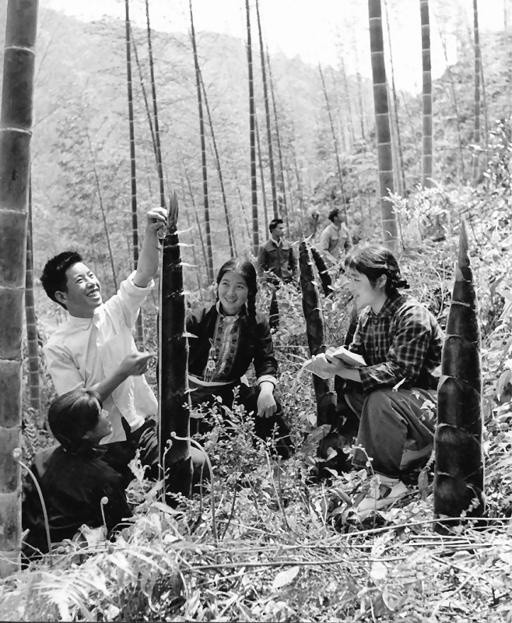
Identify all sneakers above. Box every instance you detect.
[341,477,408,520]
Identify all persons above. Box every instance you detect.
[312,245,446,512]
[41,207,211,500]
[320,207,353,265]
[257,219,298,283]
[185,257,296,460]
[21,389,133,569]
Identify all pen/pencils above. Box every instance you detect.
[337,345,348,349]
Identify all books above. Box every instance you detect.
[302,348,367,380]
